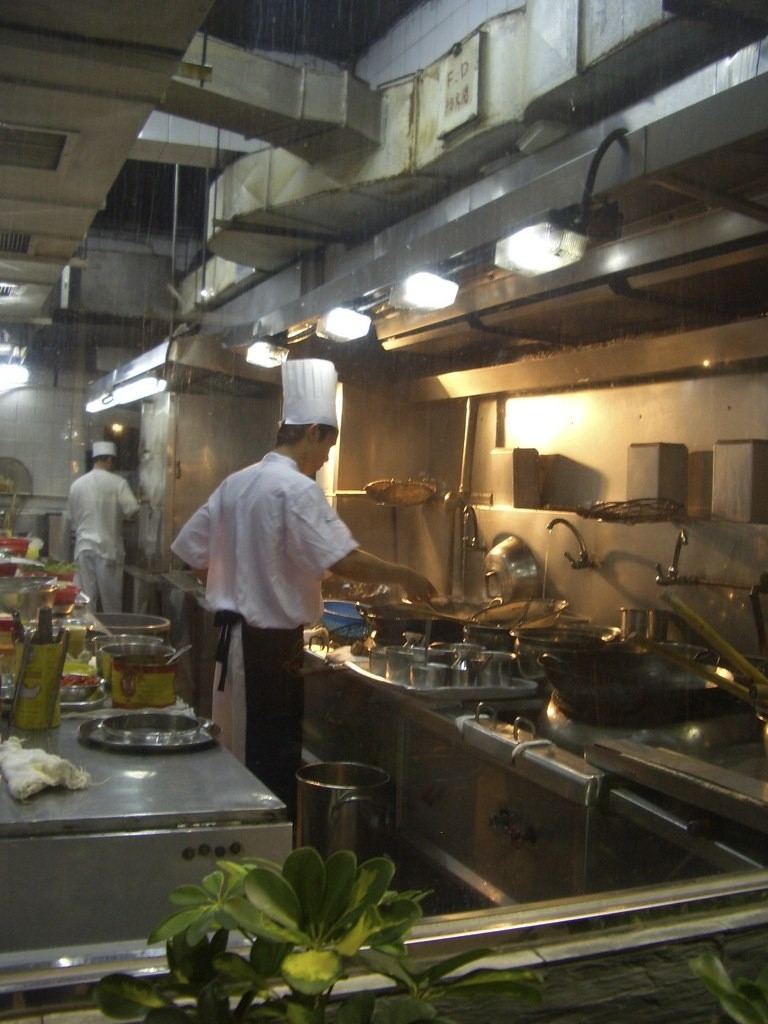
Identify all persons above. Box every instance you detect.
[169,358,437,852]
[65,442,140,613]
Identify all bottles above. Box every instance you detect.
[14,635,63,732]
[93,635,179,707]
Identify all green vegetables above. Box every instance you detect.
[0,550,80,574]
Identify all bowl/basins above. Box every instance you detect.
[368,643,518,689]
[0,536,75,582]
[481,531,538,605]
[58,675,107,700]
[0,575,79,618]
[319,597,365,635]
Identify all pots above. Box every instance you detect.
[461,596,621,679]
[346,595,502,640]
[535,641,719,721]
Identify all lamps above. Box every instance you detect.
[494,203,591,277]
[245,330,290,369]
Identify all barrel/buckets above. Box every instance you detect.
[295,761,391,866]
[91,610,171,646]
[619,607,669,643]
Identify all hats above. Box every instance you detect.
[279,359,339,428]
[92,442,116,458]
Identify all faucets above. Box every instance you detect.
[546,518,594,570]
[138,484,151,505]
[654,528,688,586]
[462,505,487,552]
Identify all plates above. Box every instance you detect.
[56,693,110,709]
[75,712,219,750]
